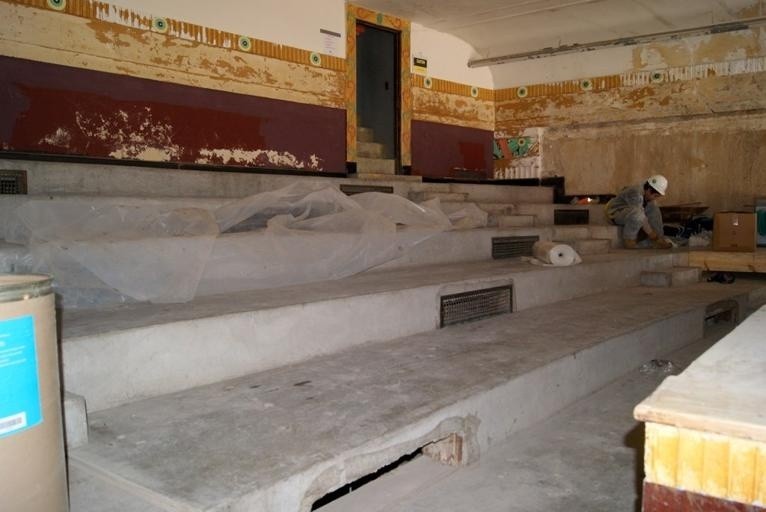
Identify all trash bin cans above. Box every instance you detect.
[756,197,766,248]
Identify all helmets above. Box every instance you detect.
[646,173,668,197]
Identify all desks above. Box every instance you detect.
[629,303,766,511]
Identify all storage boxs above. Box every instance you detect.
[712,210,758,253]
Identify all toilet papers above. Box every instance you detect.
[533,240,581,266]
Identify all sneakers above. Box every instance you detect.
[622,239,640,250]
[651,239,672,249]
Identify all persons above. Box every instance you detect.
[603,174,677,249]
